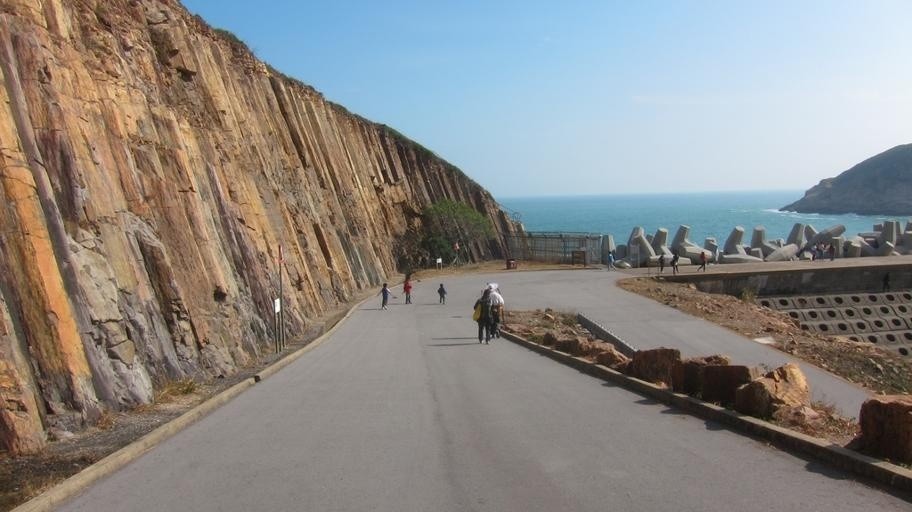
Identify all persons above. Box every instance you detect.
[437,283,447,304]
[671,256,679,275]
[473,281,506,344]
[659,251,666,273]
[402,279,413,304]
[607,251,616,272]
[810,241,835,262]
[375,282,393,310]
[696,251,707,272]
[882,271,890,292]
[673,253,681,273]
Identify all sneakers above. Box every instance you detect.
[479,330,500,345]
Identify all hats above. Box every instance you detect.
[486,283,498,291]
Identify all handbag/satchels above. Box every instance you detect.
[472,304,482,321]
